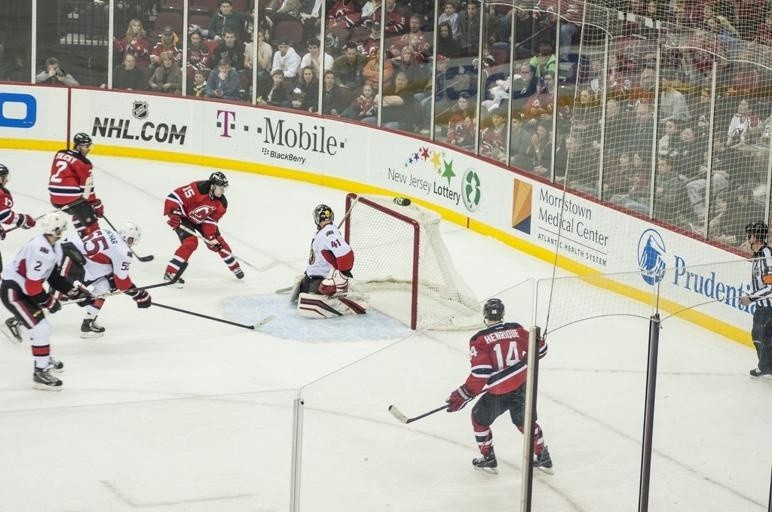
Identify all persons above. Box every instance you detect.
[294,202,370,318]
[1,130,155,391]
[445,298,554,468]
[162,171,245,283]
[738,220,771,378]
[0,0,771,254]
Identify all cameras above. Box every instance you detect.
[56,67,64,77]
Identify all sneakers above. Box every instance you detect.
[750,368,772,377]
[49,356,63,369]
[164,273,185,283]
[533,448,552,468]
[6,318,23,342]
[33,371,62,386]
[81,320,105,333]
[234,267,244,279]
[472,454,498,467]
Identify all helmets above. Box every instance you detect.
[314,205,334,227]
[118,221,141,248]
[0,164,9,176]
[209,172,228,186]
[74,132,92,146]
[40,213,69,239]
[746,221,768,242]
[483,299,504,321]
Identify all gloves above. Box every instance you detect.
[93,204,104,218]
[446,384,474,412]
[132,290,151,308]
[167,212,181,228]
[71,287,95,307]
[206,236,223,252]
[45,296,61,313]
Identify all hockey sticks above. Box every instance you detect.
[150,301,276,330]
[59,263,188,306]
[276,194,361,292]
[179,224,285,271]
[388,390,487,423]
[5,176,92,234]
[102,215,154,262]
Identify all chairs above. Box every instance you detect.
[152,0,380,91]
